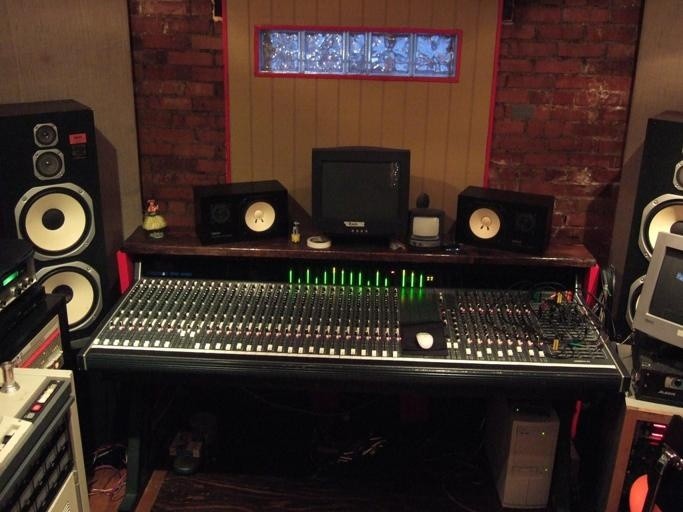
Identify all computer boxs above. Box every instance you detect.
[486,400,561,509]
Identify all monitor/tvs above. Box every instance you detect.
[633,233,683,348]
[312,147,410,235]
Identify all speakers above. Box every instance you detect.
[0,99,119,351]
[194,180,288,245]
[456,186,555,256]
[618,110,683,340]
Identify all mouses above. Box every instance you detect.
[415,332,434,349]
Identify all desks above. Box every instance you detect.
[600,380,683,512]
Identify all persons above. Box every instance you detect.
[142,199,169,238]
